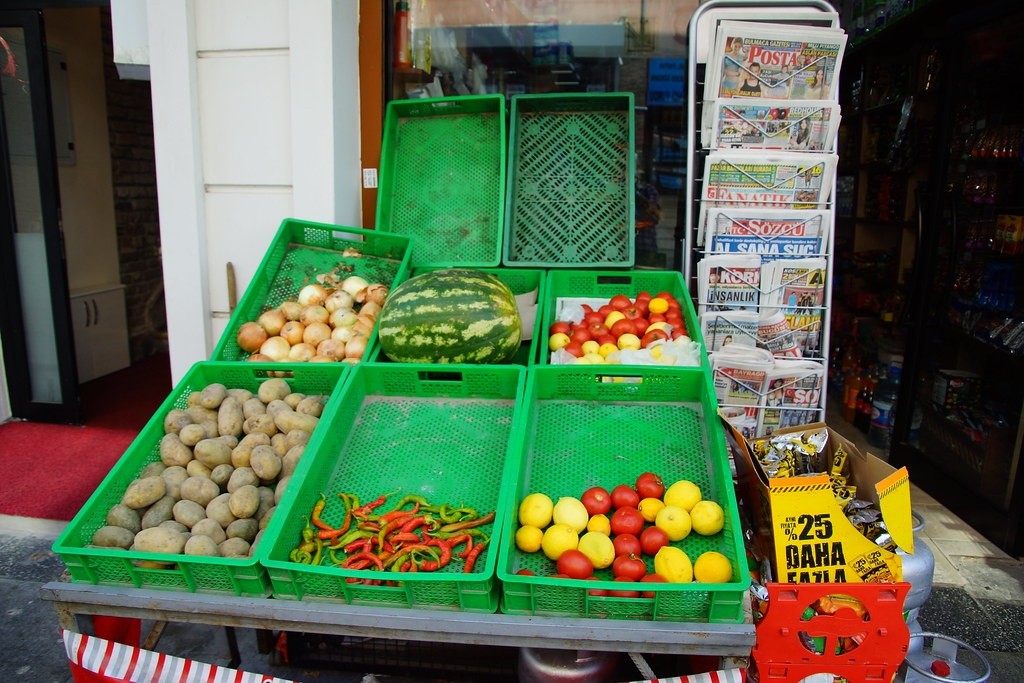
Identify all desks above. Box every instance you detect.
[39,568,756,683]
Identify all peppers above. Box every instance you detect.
[289,494,496,588]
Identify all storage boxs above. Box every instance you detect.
[718,412,914,614]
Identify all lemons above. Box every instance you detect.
[551,297,691,383]
[516,480,733,584]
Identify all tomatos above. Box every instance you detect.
[548,292,690,348]
[516,473,670,598]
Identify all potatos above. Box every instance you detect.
[92,378,323,569]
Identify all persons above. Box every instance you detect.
[710,37,829,100]
[767,378,784,406]
[710,269,720,283]
[787,292,816,315]
[794,118,810,149]
[735,426,755,439]
[723,335,732,346]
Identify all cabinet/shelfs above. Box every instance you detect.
[0,8,130,386]
[828,0,1024,563]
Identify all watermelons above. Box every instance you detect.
[378,268,522,366]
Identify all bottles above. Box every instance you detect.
[830,341,902,450]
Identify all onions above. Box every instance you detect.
[236,274,388,377]
